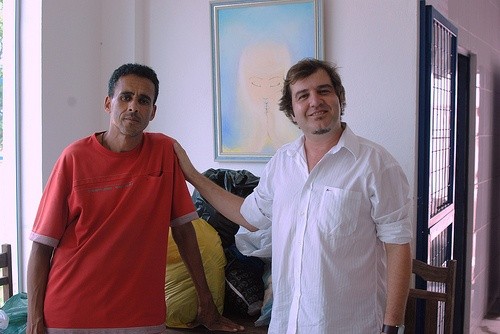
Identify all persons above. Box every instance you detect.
[173,56,414,334]
[26,64,245,334]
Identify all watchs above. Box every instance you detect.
[382,324,405,334]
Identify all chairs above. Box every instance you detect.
[405,259,457,334]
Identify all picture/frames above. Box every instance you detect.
[209,0,326,162]
